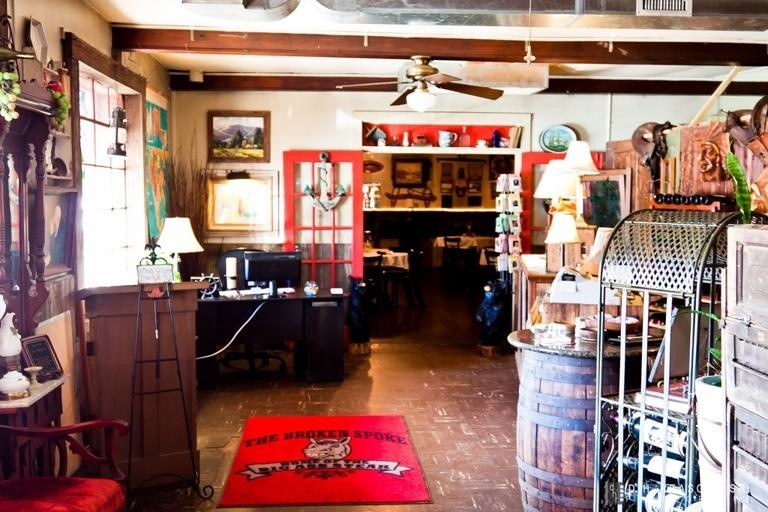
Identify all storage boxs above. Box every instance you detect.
[724,224,767,511]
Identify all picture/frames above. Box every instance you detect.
[20,332,64,383]
[391,158,427,189]
[205,107,272,164]
[199,169,282,237]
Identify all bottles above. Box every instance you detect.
[601,410,700,512]
[403,131,410,147]
[458,126,471,146]
[304,281,319,296]
[362,231,374,253]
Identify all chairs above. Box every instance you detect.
[0,414,133,512]
[363,230,501,316]
[212,248,292,373]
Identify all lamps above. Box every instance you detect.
[530,159,584,214]
[154,216,206,275]
[404,84,438,114]
[544,208,582,273]
[561,139,604,177]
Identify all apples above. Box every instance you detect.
[49,80,63,92]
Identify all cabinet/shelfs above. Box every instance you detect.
[69,281,215,491]
[0,44,71,480]
[593,210,765,512]
[683,115,767,215]
[605,123,683,258]
[517,254,619,337]
[37,124,75,284]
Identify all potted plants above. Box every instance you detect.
[689,144,756,510]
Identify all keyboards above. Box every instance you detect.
[239,286,297,297]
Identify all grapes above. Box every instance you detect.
[48,89,71,133]
[0,70,21,123]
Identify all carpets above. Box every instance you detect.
[216,412,437,508]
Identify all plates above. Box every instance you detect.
[476,145,489,148]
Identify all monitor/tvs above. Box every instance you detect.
[243,250,303,298]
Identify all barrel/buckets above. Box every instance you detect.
[694,375,725,512]
[514,349,627,512]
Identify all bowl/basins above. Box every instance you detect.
[580,327,608,341]
[604,315,640,332]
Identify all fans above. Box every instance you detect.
[335,55,503,108]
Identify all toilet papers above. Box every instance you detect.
[225,256,239,289]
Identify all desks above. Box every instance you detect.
[190,288,353,387]
[505,320,661,511]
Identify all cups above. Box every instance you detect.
[477,139,489,145]
[439,130,458,147]
